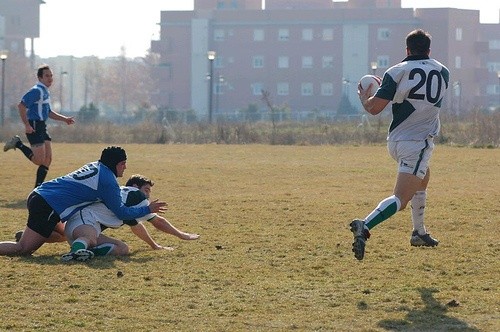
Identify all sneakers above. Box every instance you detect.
[61,249,95,261]
[15,230,23,241]
[350,219,371,260]
[4,135,22,152]
[411,230,438,247]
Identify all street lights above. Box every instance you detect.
[0,50,9,142]
[207,50,215,121]
[343,78,351,99]
[371,62,378,75]
[60,72,67,115]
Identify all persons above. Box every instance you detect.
[348,28,449,260]
[3,66,75,188]
[0,146,167,256]
[62,174,199,262]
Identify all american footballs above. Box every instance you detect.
[359,74,380,98]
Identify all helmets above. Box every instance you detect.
[98,146,127,177]
